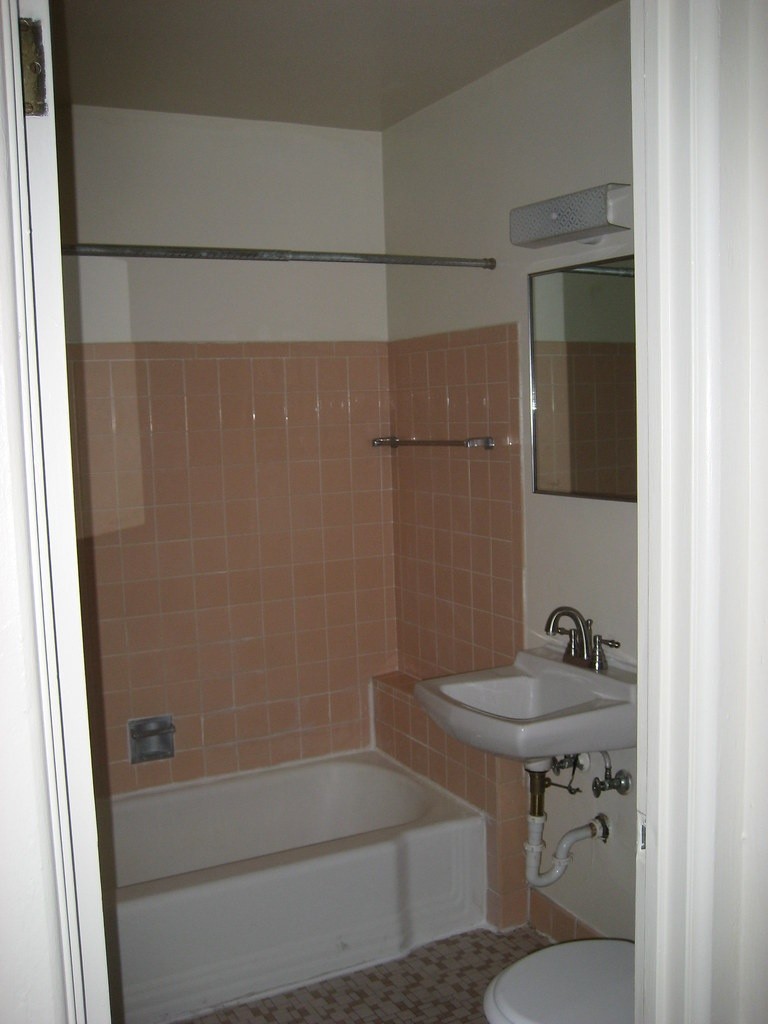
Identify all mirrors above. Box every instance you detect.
[528,254,638,505]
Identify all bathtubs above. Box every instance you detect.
[109,746,486,1024]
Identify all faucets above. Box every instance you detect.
[542,606,621,674]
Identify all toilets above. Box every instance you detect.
[487,939,634,1024]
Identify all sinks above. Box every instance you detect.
[414,645,637,762]
